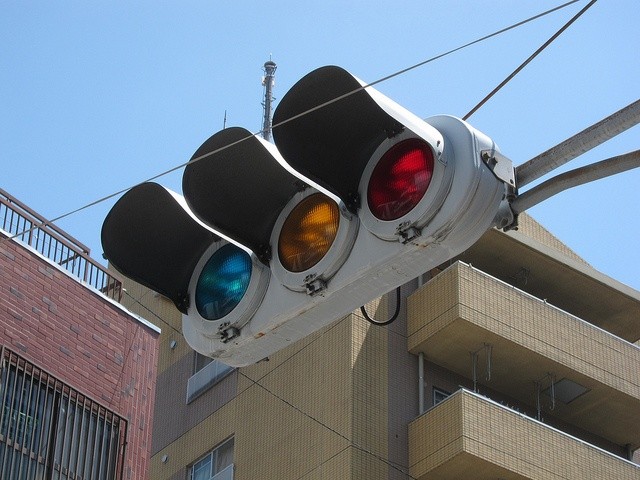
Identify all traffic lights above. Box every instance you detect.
[100,64,515,370]
